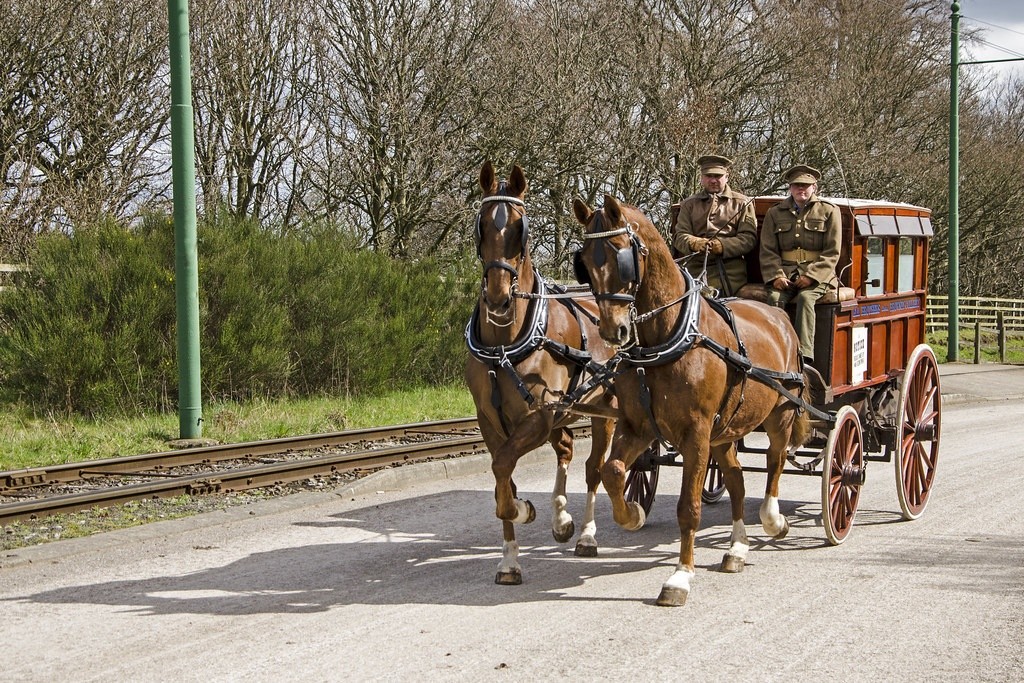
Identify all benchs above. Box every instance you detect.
[733,283,855,387]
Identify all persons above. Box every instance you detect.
[759,166,842,365]
[674,155,758,298]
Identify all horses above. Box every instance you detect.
[462,159,812,608]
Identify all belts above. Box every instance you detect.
[781,248,821,264]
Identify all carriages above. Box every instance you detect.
[461,159,944,608]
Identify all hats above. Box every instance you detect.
[785,164,821,184]
[697,156,732,175]
